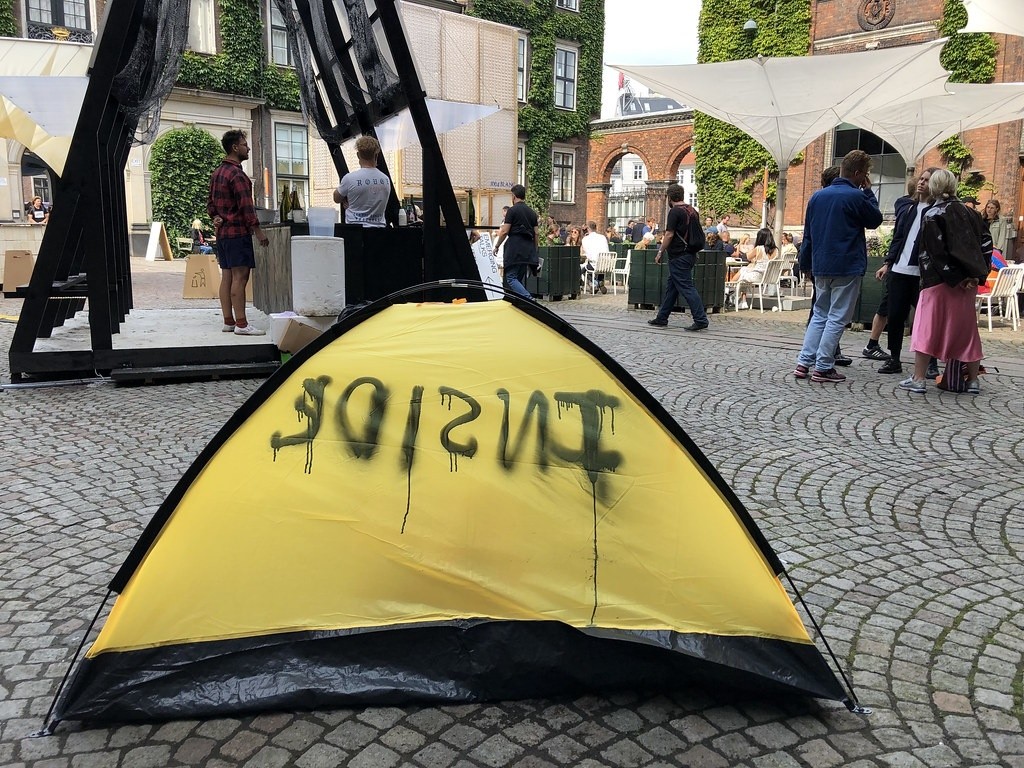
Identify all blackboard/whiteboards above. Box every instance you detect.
[146,222,173,262]
[467,232,505,300]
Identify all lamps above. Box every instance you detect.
[970,170,987,176]
[743,19,758,30]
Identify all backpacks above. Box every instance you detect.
[675,206,705,255]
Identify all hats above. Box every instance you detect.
[962,197,981,205]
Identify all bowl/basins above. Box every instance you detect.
[255,210,276,222]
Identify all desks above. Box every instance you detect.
[726,261,749,281]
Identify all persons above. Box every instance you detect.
[794,150,1007,394]
[493,184,540,303]
[333,136,391,229]
[192,218,213,254]
[206,130,269,336]
[409,205,423,222]
[538,215,667,295]
[28,195,49,225]
[500,206,510,225]
[648,184,709,331]
[702,215,797,310]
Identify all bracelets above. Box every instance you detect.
[495,246,499,248]
[882,261,890,266]
[658,250,663,253]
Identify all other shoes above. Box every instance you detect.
[591,289,599,294]
[599,281,607,294]
[738,301,748,310]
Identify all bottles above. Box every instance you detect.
[280,183,305,222]
[399,194,417,227]
[468,190,475,226]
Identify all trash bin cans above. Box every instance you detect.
[522,246,580,301]
[628,248,726,312]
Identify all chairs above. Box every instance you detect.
[976,259,1024,332]
[735,253,805,313]
[584,249,617,295]
[610,249,631,295]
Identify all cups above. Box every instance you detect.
[293,210,306,223]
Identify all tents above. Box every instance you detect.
[30,279,872,738]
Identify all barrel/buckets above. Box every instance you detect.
[307,206,335,236]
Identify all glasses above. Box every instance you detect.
[861,172,869,176]
[571,231,576,234]
[240,142,248,148]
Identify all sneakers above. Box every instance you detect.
[234,324,266,336]
[222,324,235,332]
[965,380,980,393]
[834,354,852,366]
[684,323,708,331]
[924,358,939,379]
[648,318,668,326]
[878,359,903,374]
[793,365,809,378]
[862,345,889,360]
[811,367,846,383]
[899,374,926,392]
